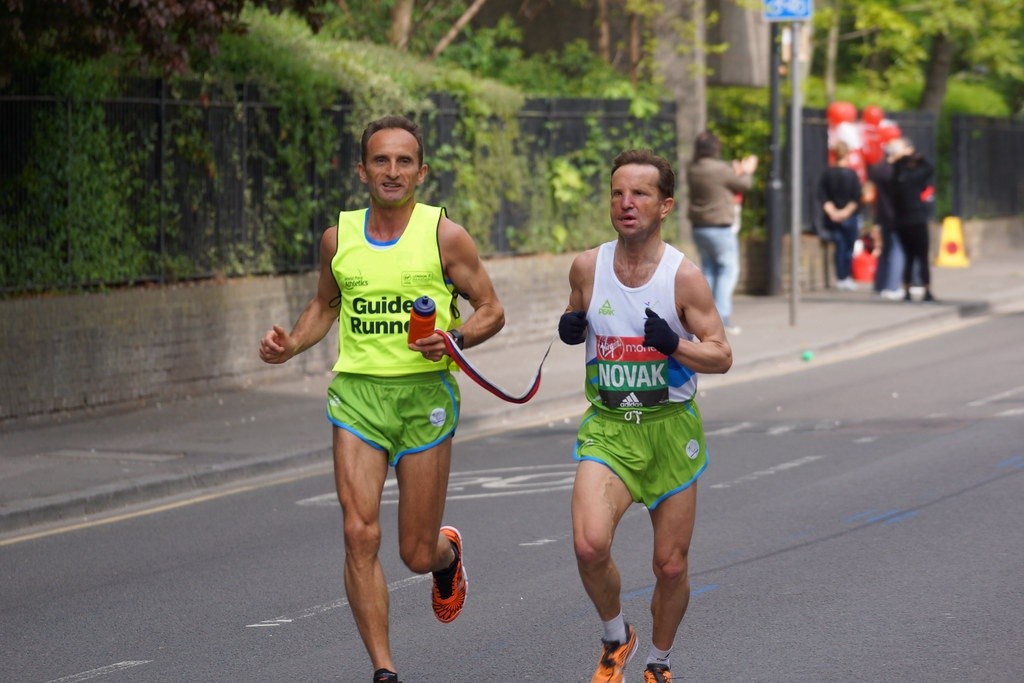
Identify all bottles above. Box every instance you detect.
[408,296,436,349]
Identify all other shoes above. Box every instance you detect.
[837,277,857,289]
[908,285,926,302]
[881,288,904,299]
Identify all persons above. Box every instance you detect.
[258,114,505,683]
[686,129,759,336]
[818,105,936,302]
[558,148,734,683]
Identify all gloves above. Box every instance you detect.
[558,311,589,345]
[641,307,679,356]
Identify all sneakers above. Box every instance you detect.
[590,621,639,683]
[431,525,468,624]
[643,659,673,683]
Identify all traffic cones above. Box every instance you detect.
[935,215,968,268]
